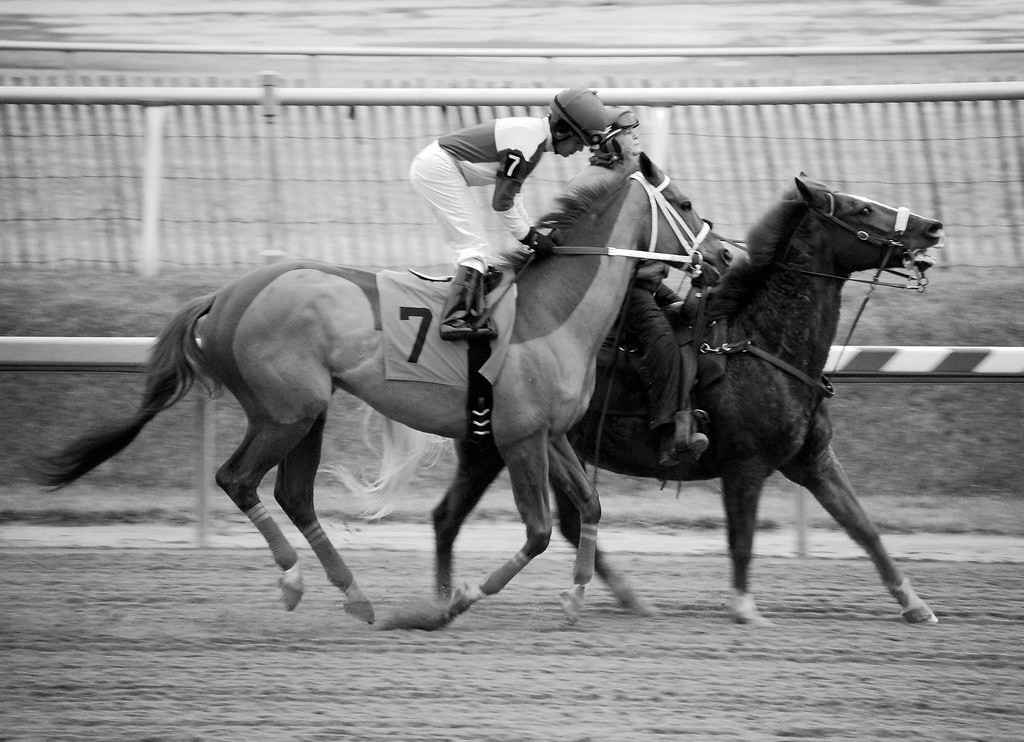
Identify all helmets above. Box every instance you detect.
[550,85,608,147]
[589,108,640,160]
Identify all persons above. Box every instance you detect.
[410,85,604,341]
[589,106,711,467]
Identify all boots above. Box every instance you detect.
[658,408,709,467]
[439,265,498,341]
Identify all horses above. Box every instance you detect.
[430,172,943,628]
[21,152,731,631]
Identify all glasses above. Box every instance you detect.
[615,111,638,127]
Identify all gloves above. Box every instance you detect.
[549,228,563,247]
[518,229,557,258]
